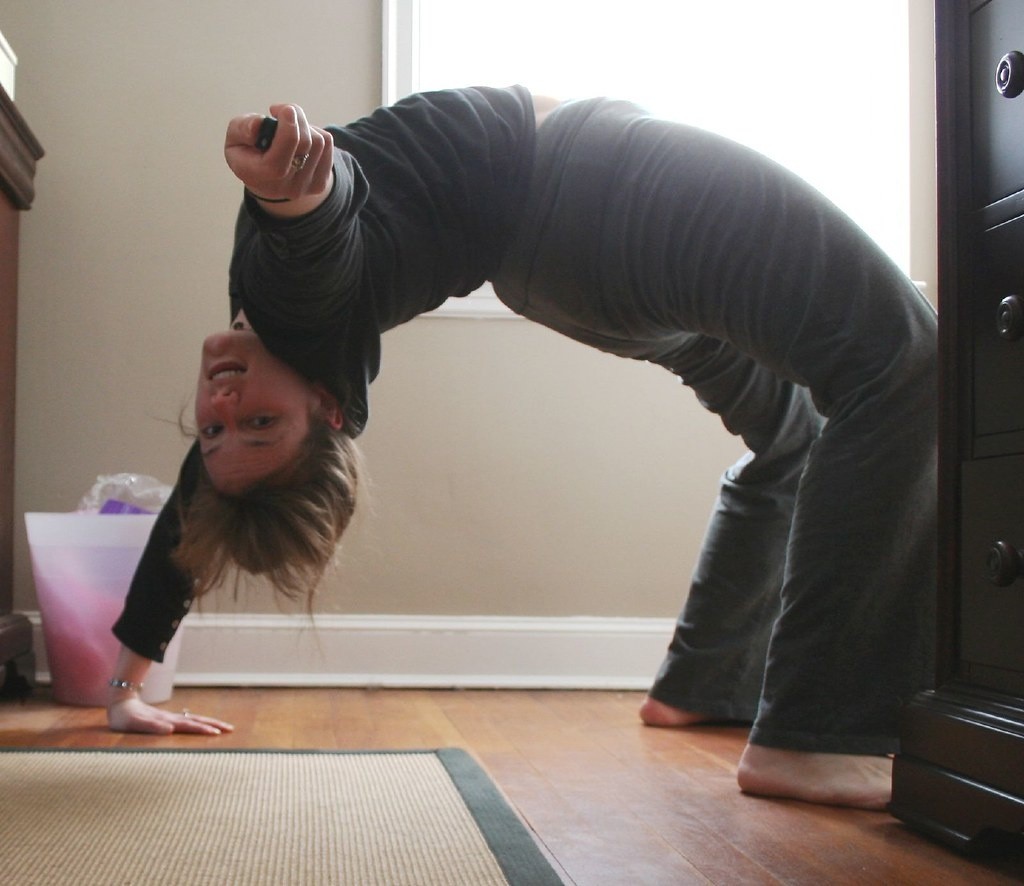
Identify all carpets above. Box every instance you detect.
[0,744,565,886]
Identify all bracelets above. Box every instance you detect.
[245,188,290,203]
[107,678,143,691]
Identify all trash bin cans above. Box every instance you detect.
[24,511,182,707]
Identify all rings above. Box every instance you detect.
[291,153,308,171]
[182,712,188,720]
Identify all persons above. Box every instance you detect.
[103,84,937,810]
